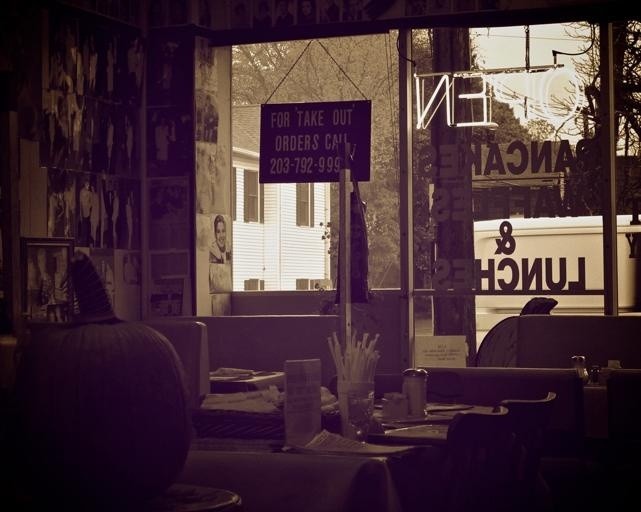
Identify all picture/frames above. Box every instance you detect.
[21,236,77,324]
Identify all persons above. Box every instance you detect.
[147,112,190,170]
[103,115,135,176]
[47,178,135,250]
[208,214,230,263]
[201,95,217,141]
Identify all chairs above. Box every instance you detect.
[425,406,514,510]
[502,393,559,511]
[149,481,243,512]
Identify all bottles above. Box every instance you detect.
[572,355,589,384]
[383,368,428,419]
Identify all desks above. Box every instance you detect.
[188,435,439,512]
[318,400,495,512]
[209,367,286,394]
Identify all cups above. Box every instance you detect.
[337,381,376,442]
[608,360,621,369]
